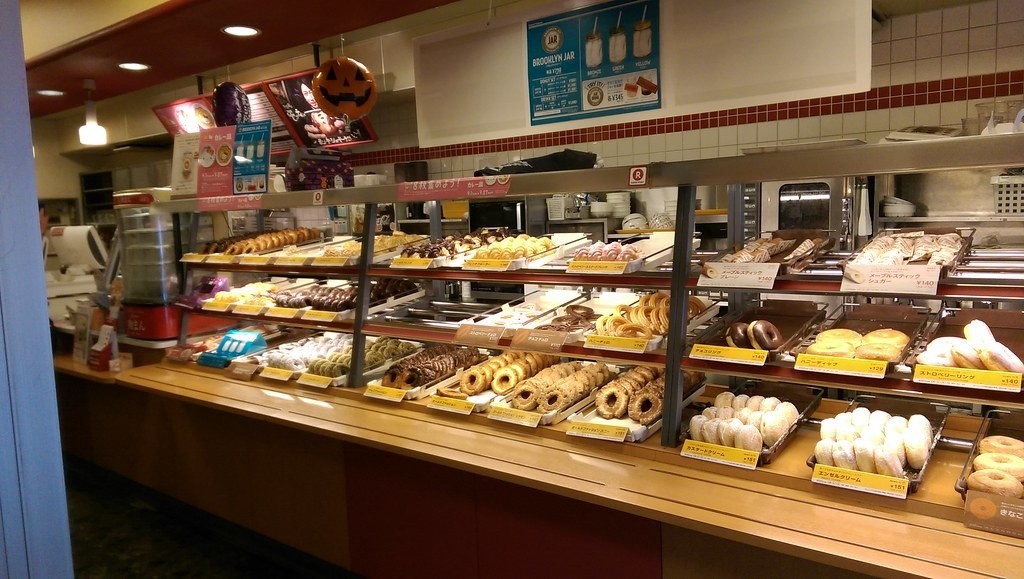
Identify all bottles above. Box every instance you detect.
[610,26,626,62]
[237,143,243,156]
[257,141,264,158]
[247,141,254,158]
[633,20,652,57]
[585,31,603,67]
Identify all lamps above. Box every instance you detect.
[78,79,108,146]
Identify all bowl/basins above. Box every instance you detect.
[883,203,916,216]
[884,196,912,204]
[591,193,673,229]
[443,201,469,218]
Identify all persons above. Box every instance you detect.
[298,79,345,140]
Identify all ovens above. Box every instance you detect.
[469,194,553,301]
[760,176,866,250]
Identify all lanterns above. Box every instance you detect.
[313,57,377,122]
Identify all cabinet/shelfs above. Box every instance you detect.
[149,130,1024,540]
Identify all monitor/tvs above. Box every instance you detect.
[50,225,109,271]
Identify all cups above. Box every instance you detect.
[974,102,1007,135]
[961,118,978,135]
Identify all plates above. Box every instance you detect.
[216,143,232,167]
[199,144,216,167]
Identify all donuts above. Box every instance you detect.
[383,226,705,424]
[690,319,1024,505]
[164,225,427,378]
